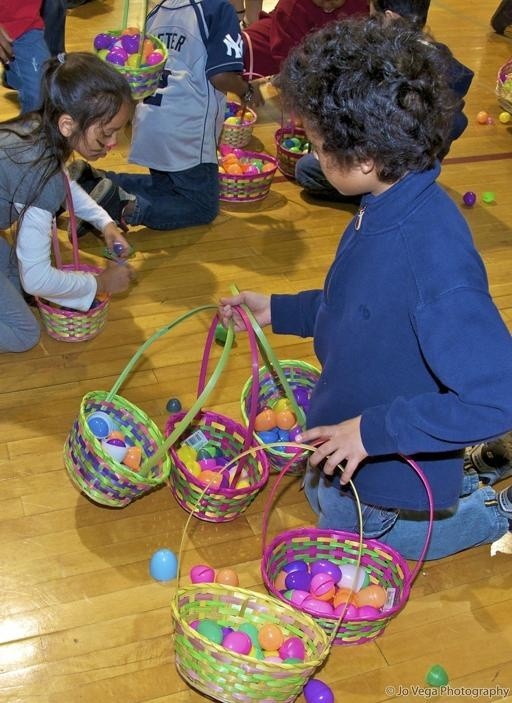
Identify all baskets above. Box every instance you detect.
[168,441,434,702]
[62,281,323,522]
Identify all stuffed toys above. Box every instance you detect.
[482,191,496,204]
[186,559,388,665]
[476,110,489,124]
[498,112,511,124]
[302,678,334,703]
[149,549,177,584]
[463,191,476,206]
[88,385,312,490]
[425,663,448,687]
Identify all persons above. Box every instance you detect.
[0,0,430,354]
[489,0,512,39]
[218,11,512,562]
